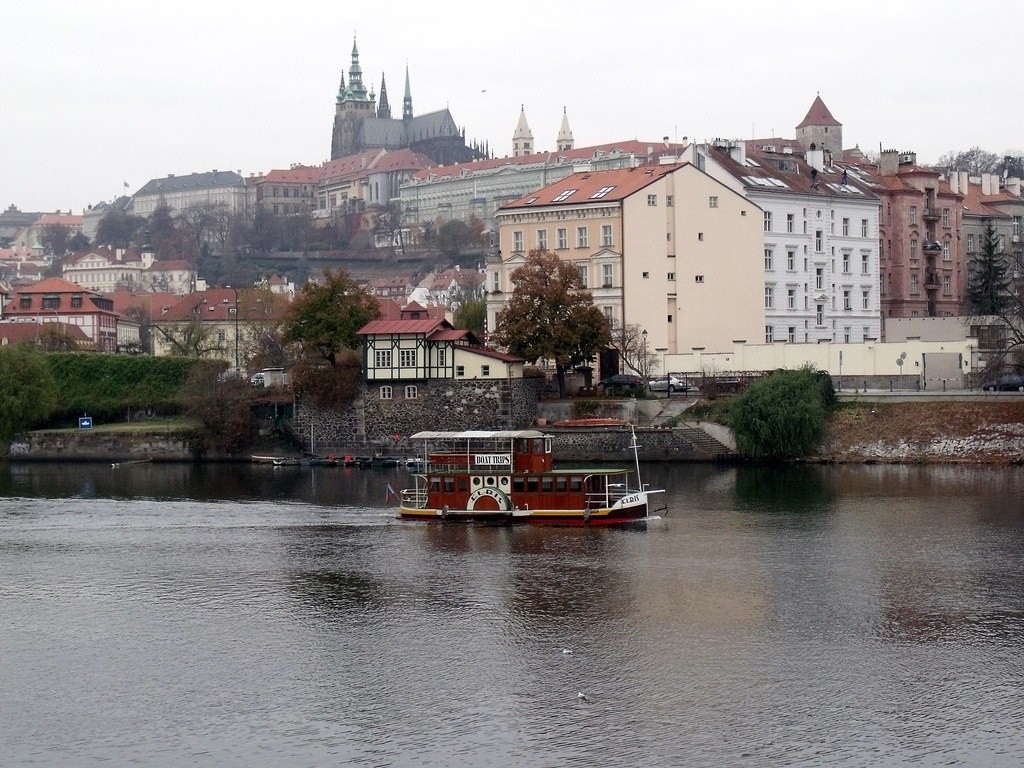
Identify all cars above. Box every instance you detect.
[598,374,644,391]
[250,372,265,387]
[983,373,1024,391]
[699,376,754,395]
[647,375,693,393]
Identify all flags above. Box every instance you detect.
[386,485,394,503]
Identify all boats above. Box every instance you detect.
[396,426,665,525]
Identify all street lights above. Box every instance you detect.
[642,329,649,397]
